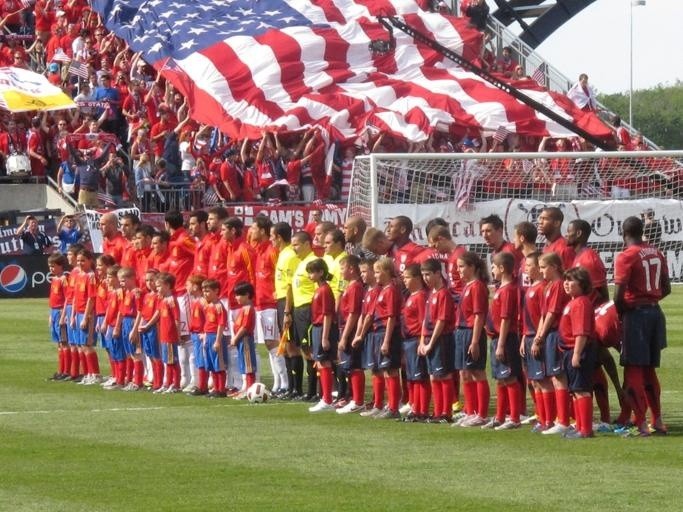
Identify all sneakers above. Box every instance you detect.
[268,388,667,438]
[48,371,250,400]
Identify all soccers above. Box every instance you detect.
[247,383,268,403]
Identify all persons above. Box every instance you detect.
[0,0,683,437]
